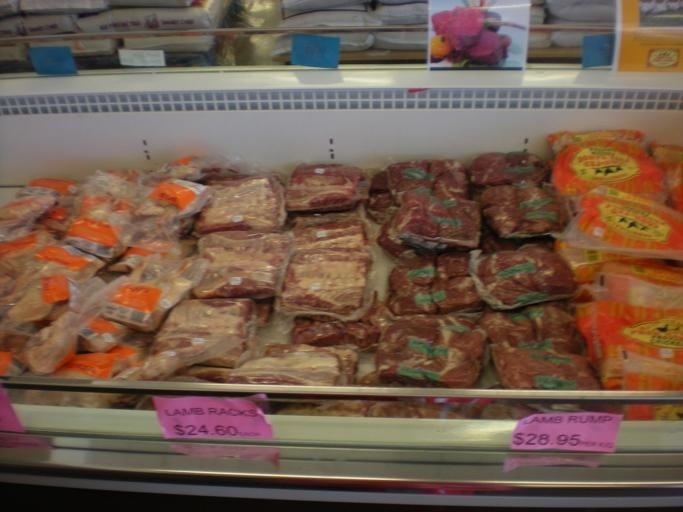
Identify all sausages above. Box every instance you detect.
[139,165,372,385]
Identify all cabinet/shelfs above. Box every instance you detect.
[0,0,683,511]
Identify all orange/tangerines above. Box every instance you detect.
[430,34,451,58]
